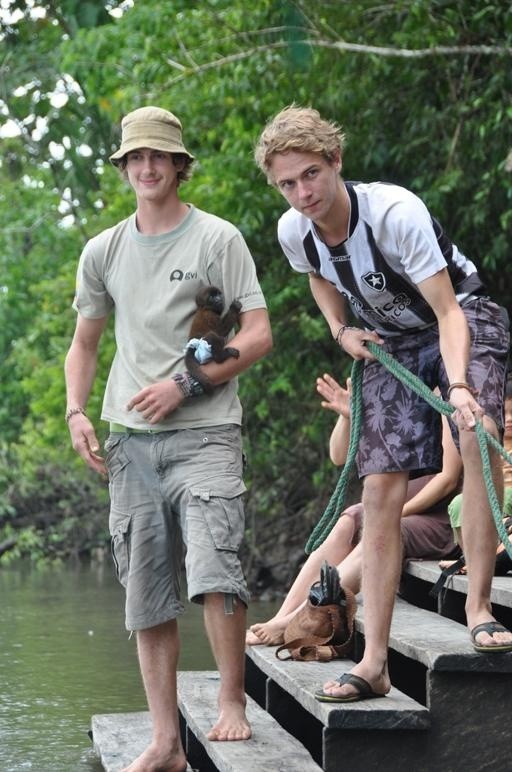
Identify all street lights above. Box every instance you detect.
[273,578,358,664]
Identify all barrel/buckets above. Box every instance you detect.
[314,671,386,703]
[468,619,512,655]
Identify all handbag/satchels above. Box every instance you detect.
[333,322,354,349]
[171,371,191,402]
[447,375,468,399]
[64,407,84,423]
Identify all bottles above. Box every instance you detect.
[109,105,195,171]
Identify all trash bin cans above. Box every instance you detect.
[187,370,204,396]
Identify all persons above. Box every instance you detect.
[60,107,276,772]
[242,371,466,645]
[439,379,511,576]
[255,100,512,703]
